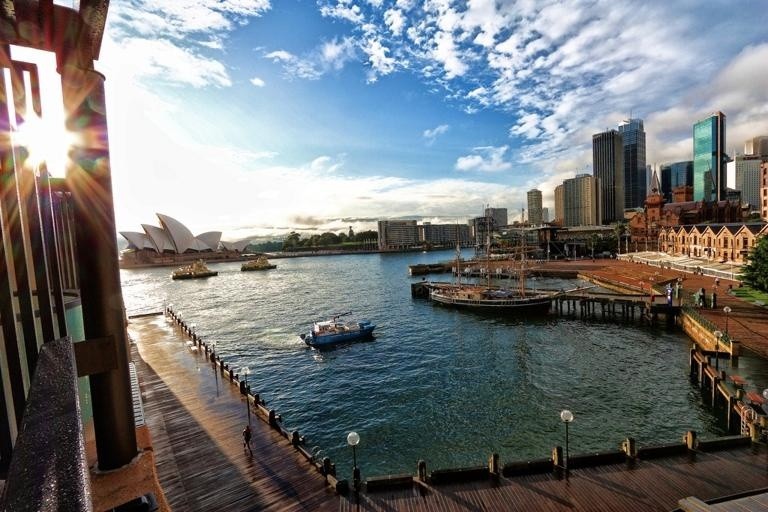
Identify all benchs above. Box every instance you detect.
[729,375,749,388]
[746,392,768,407]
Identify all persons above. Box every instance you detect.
[242,425,253,456]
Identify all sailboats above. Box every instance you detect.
[431,208,552,312]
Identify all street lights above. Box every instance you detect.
[210,338,217,382]
[713,330,723,370]
[724,306,731,336]
[560,409,573,469]
[347,431,360,512]
[638,261,683,300]
[240,366,252,425]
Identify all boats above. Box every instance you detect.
[173,259,218,280]
[300,311,375,346]
[241,256,277,271]
